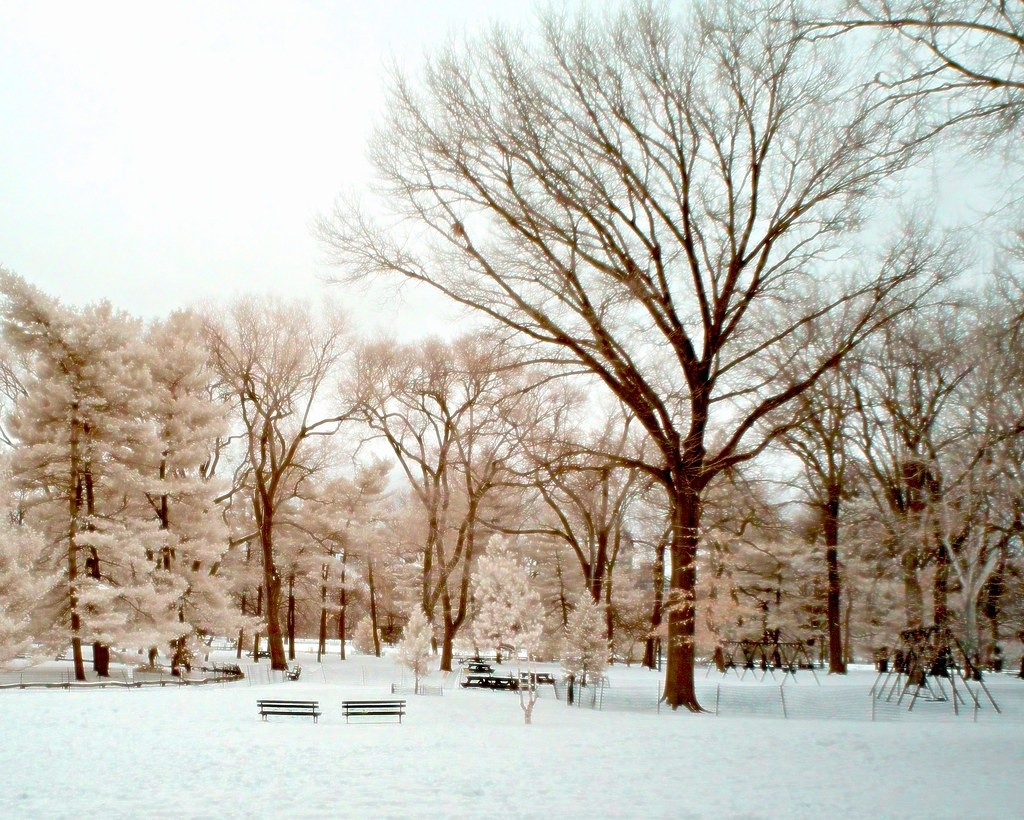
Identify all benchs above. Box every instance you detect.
[341,700,407,724]
[246,651,271,659]
[256,699,322,723]
[286,666,302,681]
[458,656,556,690]
